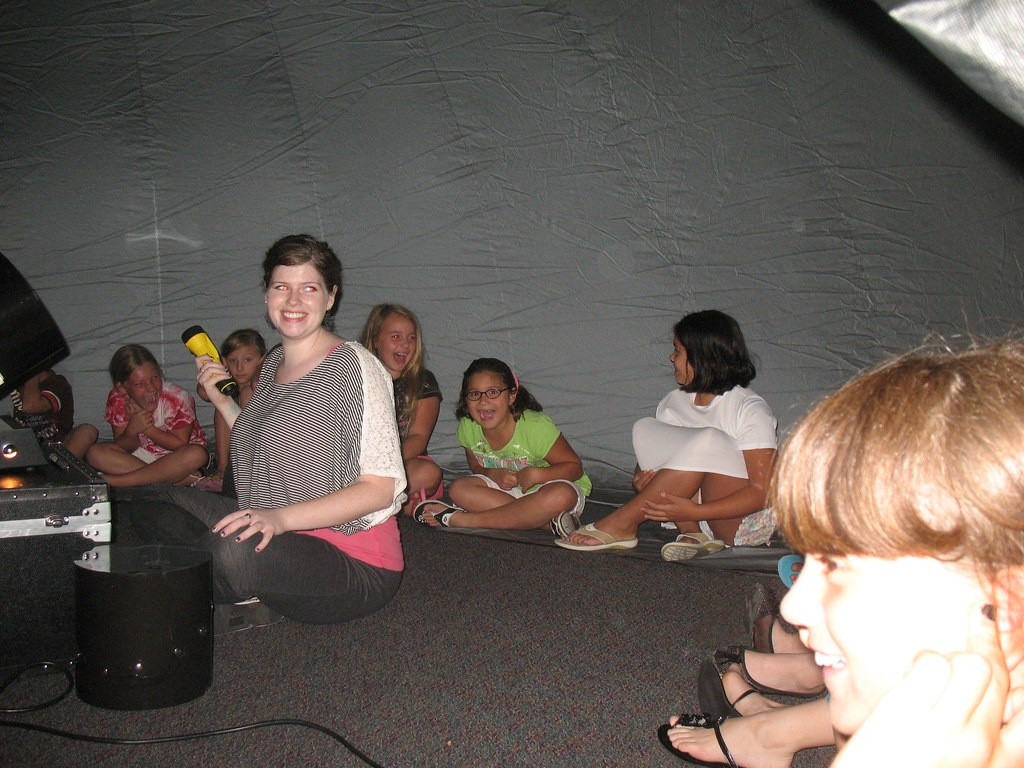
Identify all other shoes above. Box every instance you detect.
[715,645,828,698]
[196,476,223,493]
[211,593,284,636]
[743,583,780,654]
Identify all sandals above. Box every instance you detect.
[399,489,426,519]
[778,556,804,589]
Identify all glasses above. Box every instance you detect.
[463,387,512,401]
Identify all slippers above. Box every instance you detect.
[413,501,467,528]
[699,656,759,724]
[554,522,638,551]
[178,472,206,488]
[550,511,581,538]
[660,533,725,561]
[658,713,742,768]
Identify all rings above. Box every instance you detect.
[245,513,251,518]
[200,368,204,373]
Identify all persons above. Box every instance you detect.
[132,235,407,637]
[196,328,268,494]
[657,343,1024,768]
[555,309,782,559]
[88,344,210,490]
[359,304,444,516]
[415,358,592,538]
[10,369,99,458]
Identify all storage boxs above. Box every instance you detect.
[0,440,112,665]
